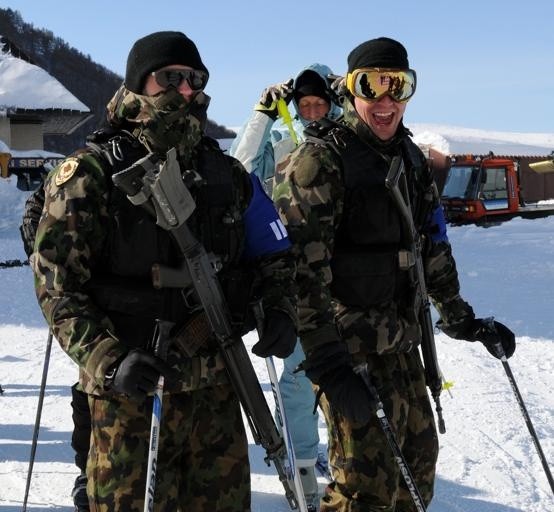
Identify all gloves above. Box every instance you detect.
[327,73,347,96]
[253,79,295,121]
[471,319,515,358]
[252,310,297,359]
[109,349,174,402]
[317,361,373,423]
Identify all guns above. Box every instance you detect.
[385,151,446,434]
[111,147,299,509]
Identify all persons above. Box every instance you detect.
[220,62,353,511]
[27,28,302,511]
[19,178,93,510]
[261,34,517,512]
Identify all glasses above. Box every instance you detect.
[149,68,209,90]
[345,67,417,104]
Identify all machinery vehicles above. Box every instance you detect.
[437,151,553,228]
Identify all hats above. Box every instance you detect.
[295,70,331,106]
[124,31,209,95]
[348,38,409,74]
